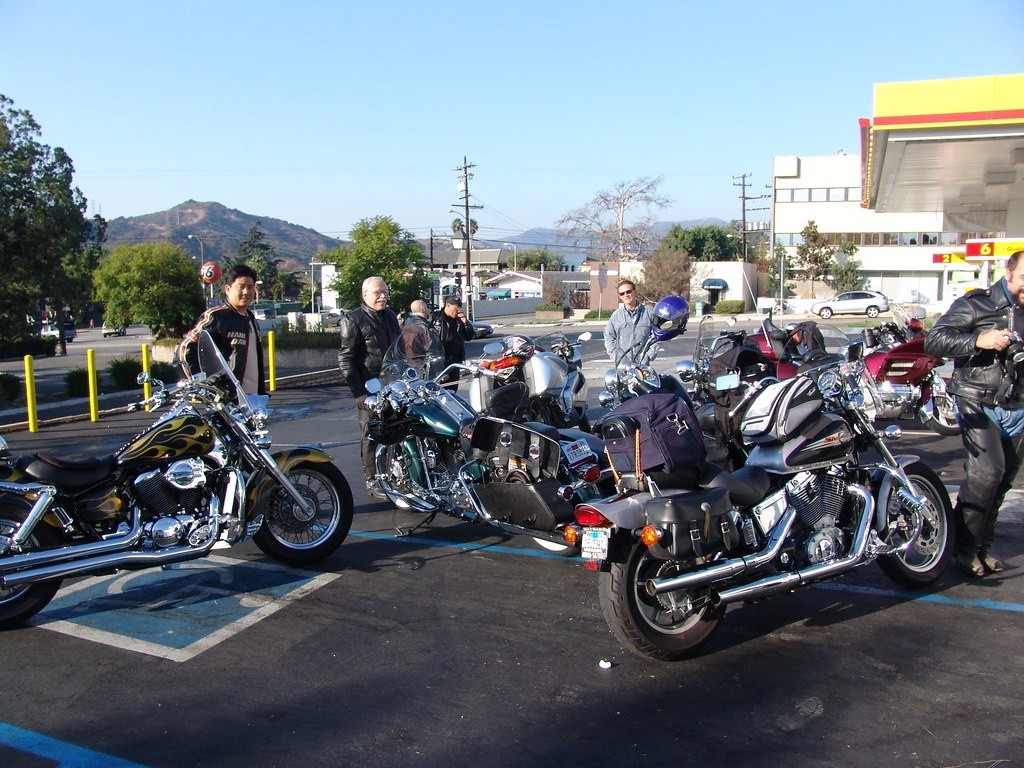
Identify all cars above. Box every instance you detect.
[40,323,77,342]
[810,290,890,319]
[101,321,127,337]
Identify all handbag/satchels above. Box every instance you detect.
[709,350,773,408]
[600,393,707,473]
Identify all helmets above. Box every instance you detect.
[652,295,690,341]
[507,334,534,356]
[368,415,411,445]
[785,323,803,344]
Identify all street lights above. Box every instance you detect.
[726,234,747,262]
[189,234,206,299]
[503,243,516,272]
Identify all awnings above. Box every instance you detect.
[486,287,511,298]
[702,278,728,290]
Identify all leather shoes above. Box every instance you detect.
[366,479,389,499]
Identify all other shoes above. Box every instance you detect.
[955,542,985,576]
[977,548,1004,571]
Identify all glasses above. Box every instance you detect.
[364,291,389,296]
[618,289,635,297]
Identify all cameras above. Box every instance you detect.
[1004,331,1024,363]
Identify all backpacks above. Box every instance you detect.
[794,320,826,355]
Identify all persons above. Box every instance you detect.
[921,249,1024,576]
[403,299,449,383]
[338,277,405,499]
[431,296,476,394]
[179,264,266,409]
[88,319,95,329]
[601,281,662,369]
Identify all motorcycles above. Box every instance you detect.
[0,330,356,629]
[575,323,955,662]
[361,307,969,561]
